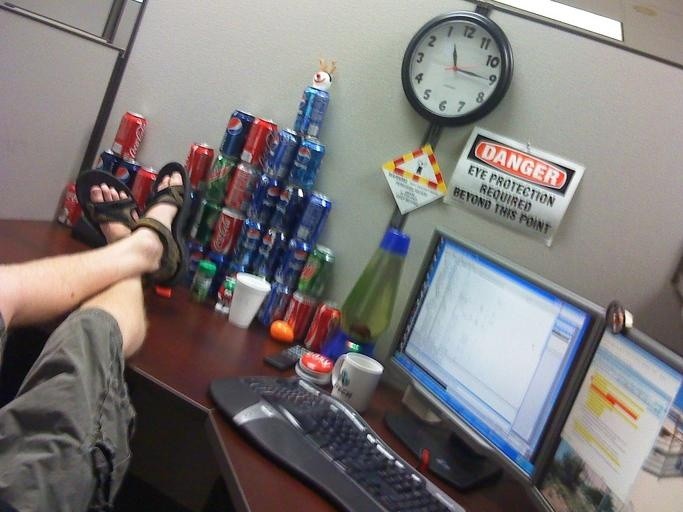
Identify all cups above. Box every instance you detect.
[226,271,272,331]
[331,352,384,414]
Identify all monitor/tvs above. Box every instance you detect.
[384,226,604,492]
[529,315,683,511]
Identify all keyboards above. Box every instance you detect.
[208,374,467,511]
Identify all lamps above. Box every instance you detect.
[602,298,635,336]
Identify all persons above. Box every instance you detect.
[0,162,187,511]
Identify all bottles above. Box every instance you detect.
[321,227,410,365]
[189,259,215,303]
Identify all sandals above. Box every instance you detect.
[75,170,141,246]
[131,162,192,287]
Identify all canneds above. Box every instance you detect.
[58,85,340,350]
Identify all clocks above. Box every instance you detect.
[402,11,515,128]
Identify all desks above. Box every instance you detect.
[1,221,547,512]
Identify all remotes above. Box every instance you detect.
[262,345,310,370]
